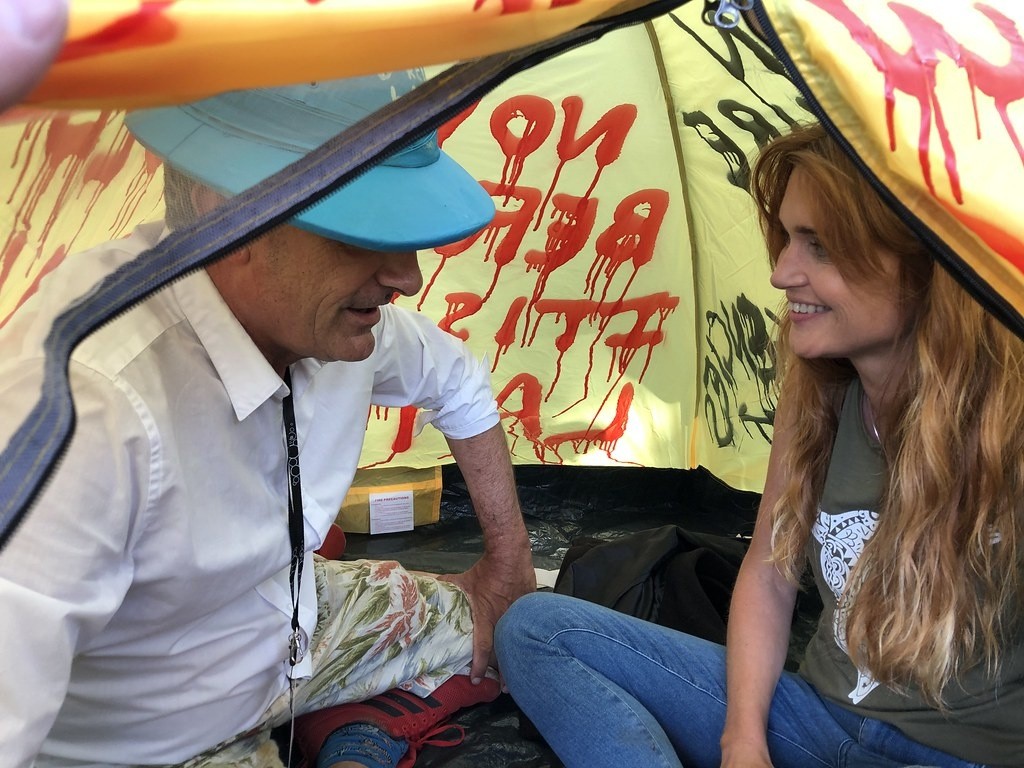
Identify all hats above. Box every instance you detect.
[122,65,494,251]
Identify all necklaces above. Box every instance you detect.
[863,394,885,453]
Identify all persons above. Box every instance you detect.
[0,67,538,768]
[492,121,1024,767]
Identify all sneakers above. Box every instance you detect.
[273,673,501,768]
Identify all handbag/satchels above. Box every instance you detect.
[517,525,823,749]
